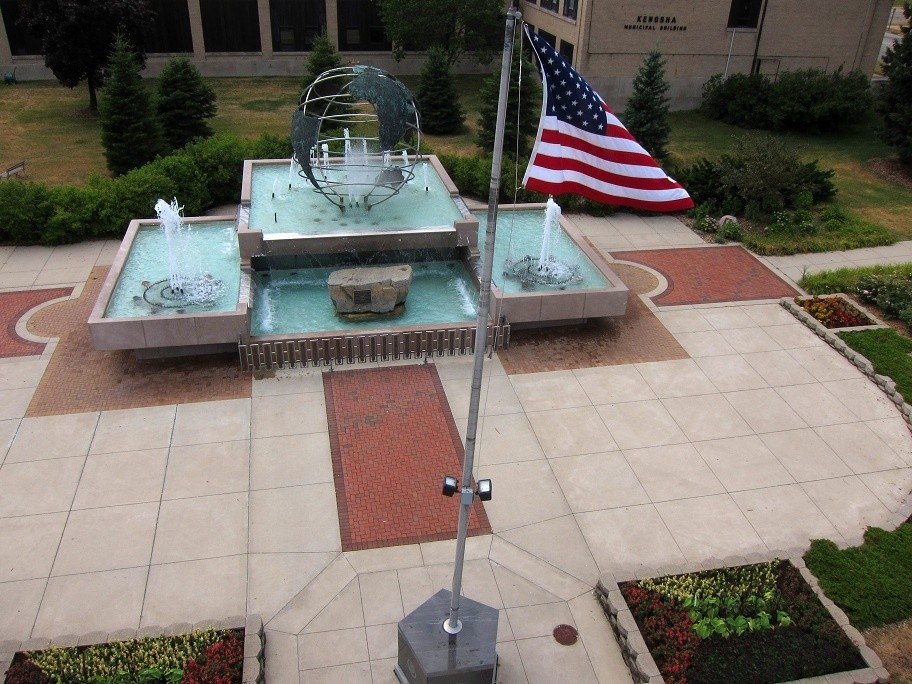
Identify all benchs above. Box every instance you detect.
[1,160,25,180]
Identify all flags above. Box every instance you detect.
[521,23,695,211]
